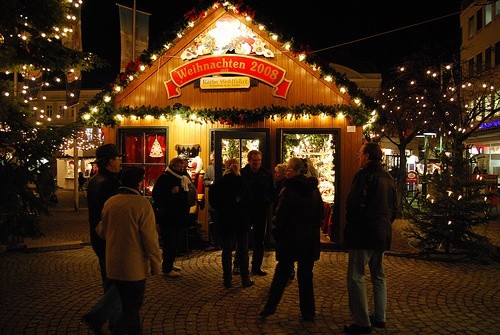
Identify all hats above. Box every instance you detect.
[90,144,128,165]
[117,166,146,180]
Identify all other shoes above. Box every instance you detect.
[172,266,181,271]
[166,270,181,278]
[345,323,373,335]
[82,313,107,335]
[370,316,386,328]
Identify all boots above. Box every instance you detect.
[232,261,241,275]
[221,273,232,288]
[241,274,255,289]
[251,262,268,276]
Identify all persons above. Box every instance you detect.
[233,150,274,275]
[150,156,198,276]
[341,143,398,335]
[97,167,161,335]
[257,157,325,321]
[80,143,123,335]
[208,159,255,288]
